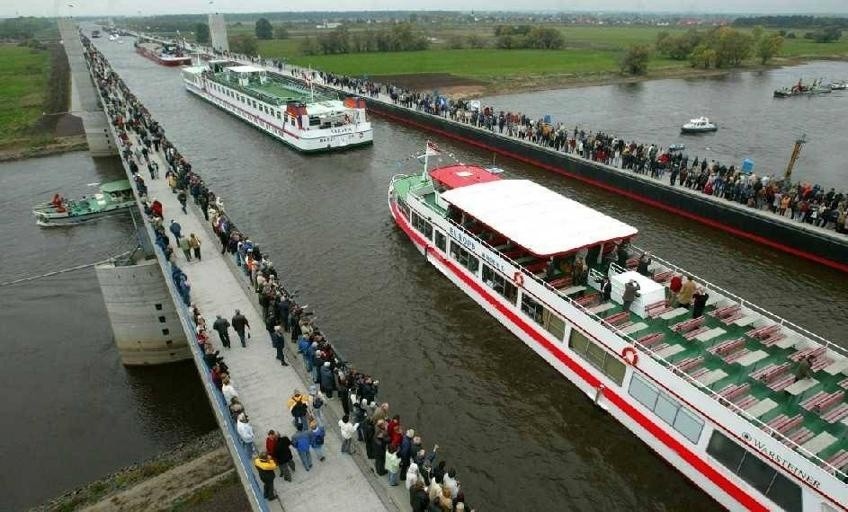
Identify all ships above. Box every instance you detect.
[388,142,847,512]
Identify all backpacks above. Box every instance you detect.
[291,396,307,418]
[315,434,324,446]
[309,391,323,408]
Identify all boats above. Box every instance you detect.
[89,26,126,45]
[773,83,831,98]
[829,79,847,92]
[179,49,375,155]
[134,37,187,68]
[681,116,719,134]
[33,178,137,230]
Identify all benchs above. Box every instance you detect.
[456,211,848,482]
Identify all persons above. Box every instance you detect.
[203,47,383,97]
[572,237,709,318]
[144,171,469,511]
[386,80,848,236]
[53,194,92,214]
[81,35,192,205]
